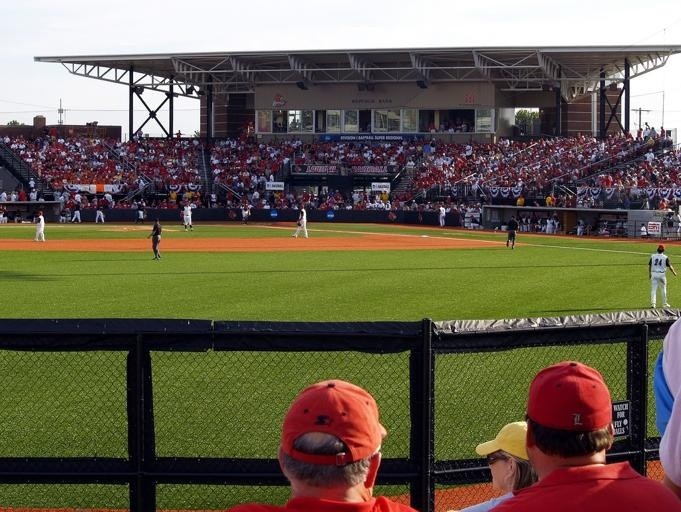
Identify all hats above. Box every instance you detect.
[277,377,388,469]
[473,417,539,465]
[658,244,665,250]
[523,357,619,433]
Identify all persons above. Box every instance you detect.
[225,379,418,512]
[659,381,681,501]
[484,360,681,512]
[654,316,681,438]
[459,420,542,512]
[648,245,677,309]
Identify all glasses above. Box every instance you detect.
[485,451,514,464]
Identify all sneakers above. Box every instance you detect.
[185,228,194,232]
[662,303,671,308]
[650,303,658,308]
